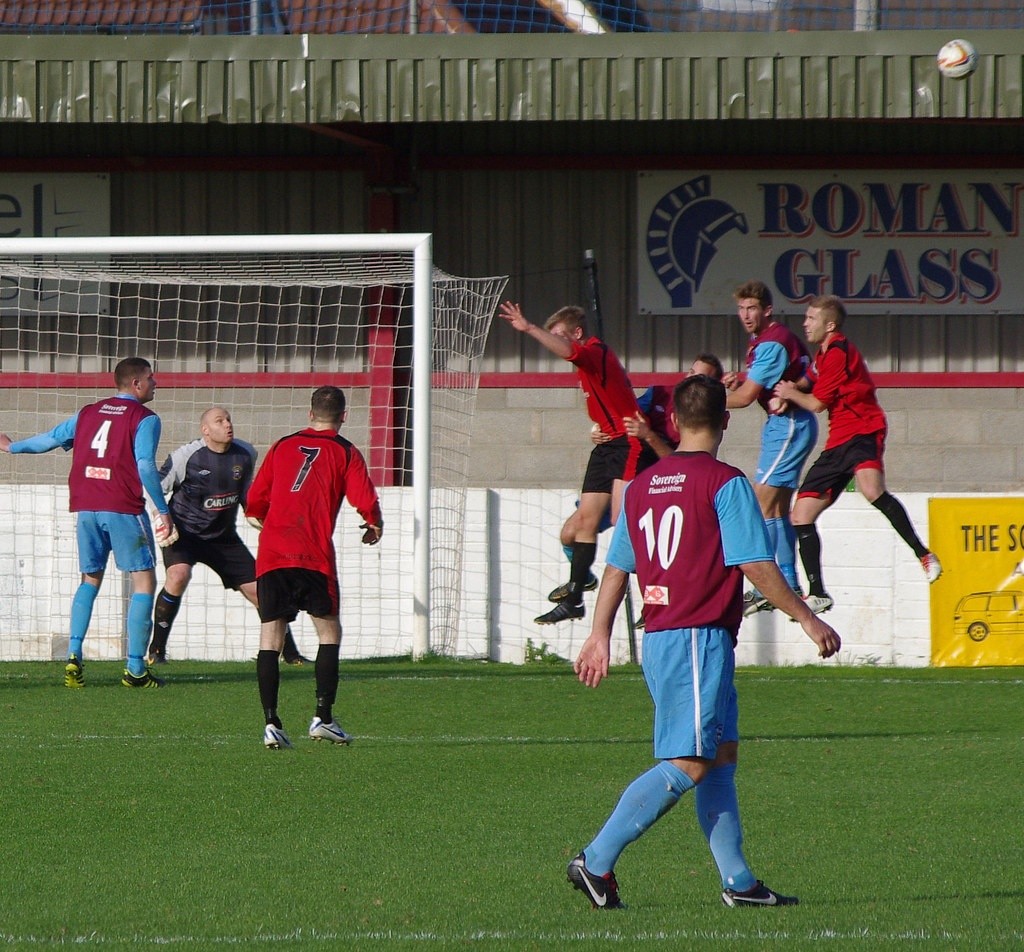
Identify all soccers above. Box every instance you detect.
[937,38,980,81]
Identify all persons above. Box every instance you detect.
[567,374,842,912]
[146,407,306,665]
[709,279,818,617]
[0,358,175,688]
[547,353,723,602]
[769,294,944,621]
[245,385,384,750]
[498,300,656,628]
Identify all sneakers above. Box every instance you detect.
[920,552,943,585]
[722,879,800,908]
[788,592,834,623]
[566,852,625,909]
[742,586,803,617]
[534,594,586,625]
[309,717,352,747]
[548,575,599,603]
[64,653,86,687]
[148,644,169,666]
[283,656,303,665]
[121,666,166,689]
[264,724,295,749]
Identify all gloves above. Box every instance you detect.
[152,512,179,547]
